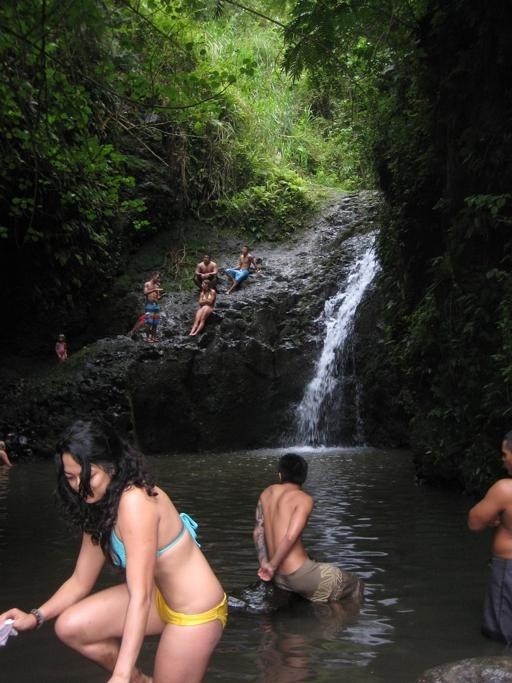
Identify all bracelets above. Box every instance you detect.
[28,609,45,632]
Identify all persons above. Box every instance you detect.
[189,279,216,335]
[1,418,229,683]
[127,309,147,339]
[470,435,512,656]
[254,453,363,605]
[193,253,219,292]
[1,441,12,467]
[55,334,69,361]
[223,245,262,295]
[144,271,162,342]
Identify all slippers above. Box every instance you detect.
[151,337,159,341]
[147,338,153,342]
